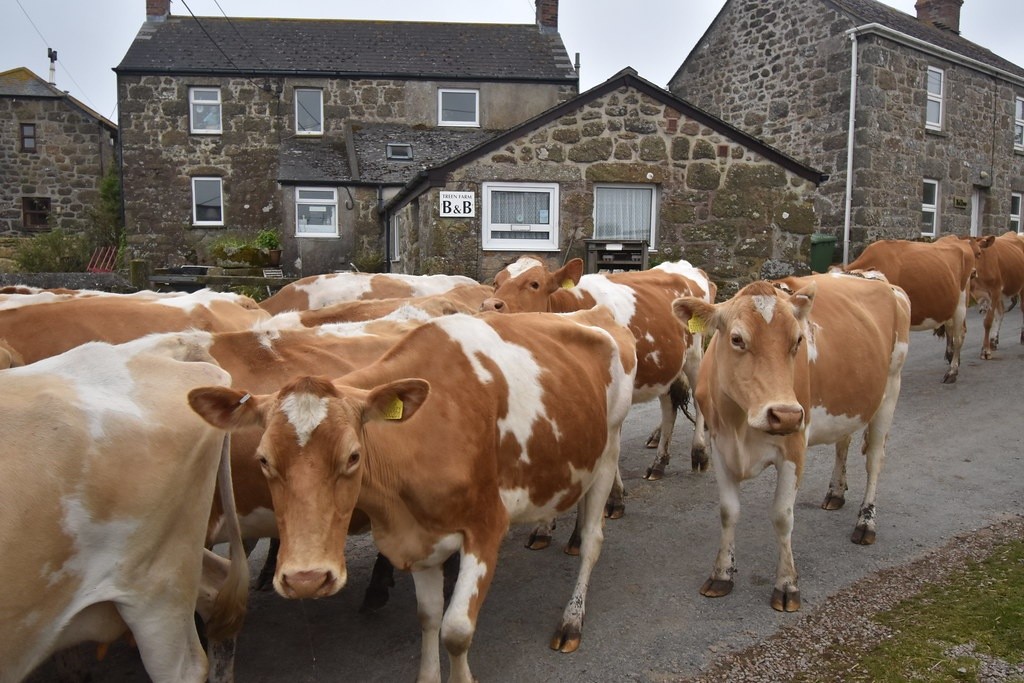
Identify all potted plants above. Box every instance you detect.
[206,228,283,268]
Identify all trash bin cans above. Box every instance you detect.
[810,234,839,274]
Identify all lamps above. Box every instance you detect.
[980,170,989,180]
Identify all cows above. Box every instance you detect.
[0,230,1024,683]
